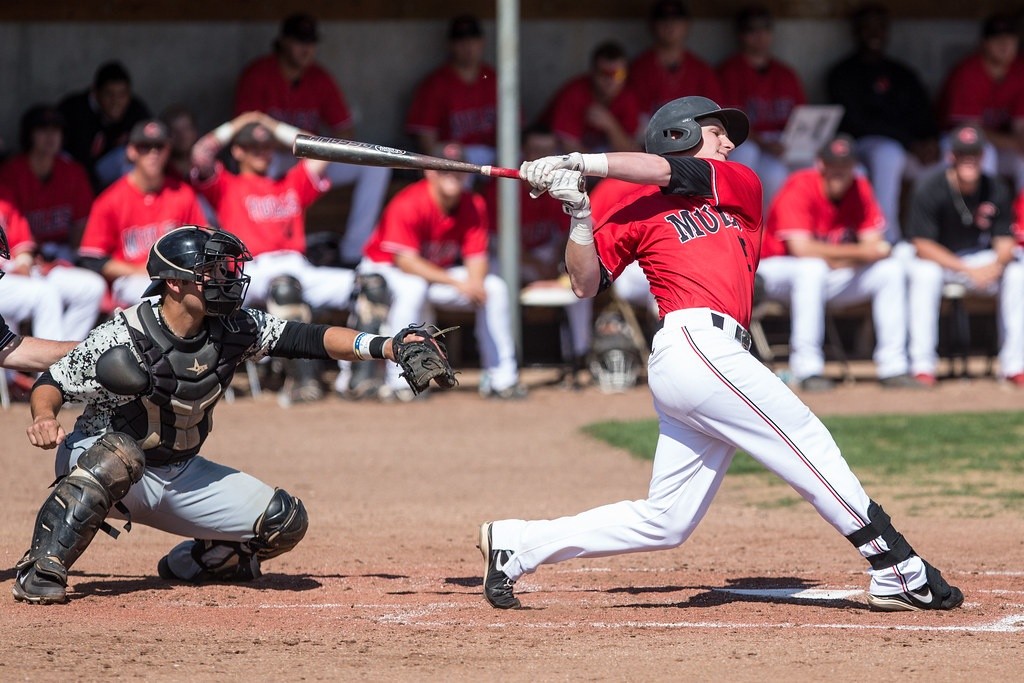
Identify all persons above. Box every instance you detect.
[480,95,965,613]
[14,226,458,603]
[0,0,1024,385]
[0,225,84,372]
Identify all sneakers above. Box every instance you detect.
[478,520,521,610]
[867,586,965,612]
[157,555,235,582]
[12,562,66,604]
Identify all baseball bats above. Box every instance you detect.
[291,133,587,193]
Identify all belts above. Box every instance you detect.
[711,313,752,350]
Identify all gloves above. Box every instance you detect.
[519,151,585,199]
[546,169,592,218]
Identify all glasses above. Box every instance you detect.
[135,143,166,155]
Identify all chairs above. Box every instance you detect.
[224,283,995,401]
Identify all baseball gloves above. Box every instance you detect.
[392,325,456,396]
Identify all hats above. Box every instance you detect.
[233,122,276,147]
[128,120,172,145]
[947,117,992,153]
[279,10,325,45]
[817,131,860,169]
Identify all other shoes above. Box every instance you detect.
[224,347,1023,404]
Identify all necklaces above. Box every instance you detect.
[157,305,182,338]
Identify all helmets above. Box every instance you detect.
[644,96,750,156]
[140,224,253,334]
[588,312,643,397]
